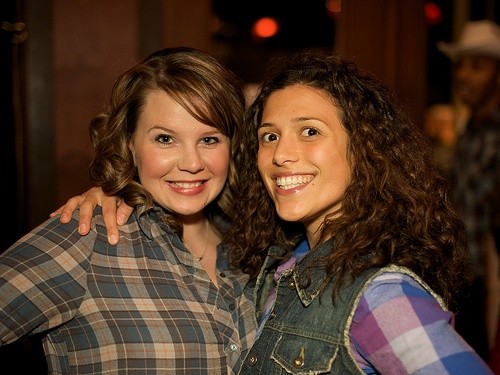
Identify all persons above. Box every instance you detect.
[0,46,261,375]
[425,21,500,374]
[49,48,494,375]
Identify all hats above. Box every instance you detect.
[438,21,499,58]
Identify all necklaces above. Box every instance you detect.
[181,217,210,261]
